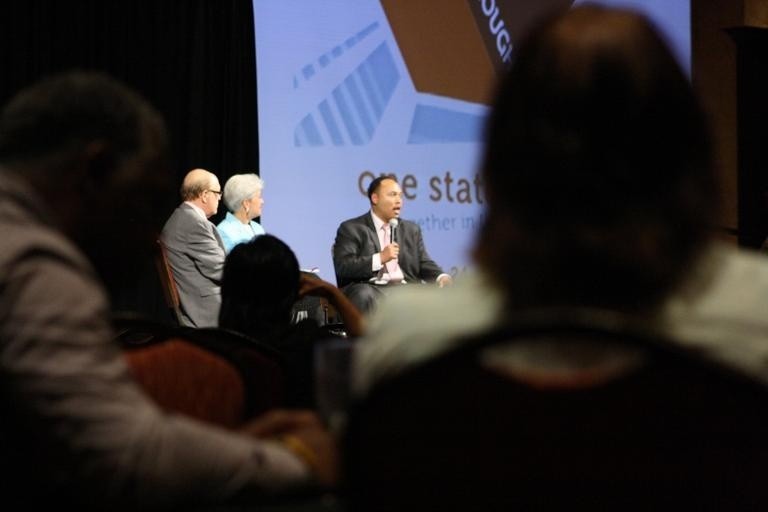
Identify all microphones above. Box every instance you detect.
[388,218,398,243]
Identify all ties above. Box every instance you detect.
[383,224,404,281]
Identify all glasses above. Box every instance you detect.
[209,187,223,195]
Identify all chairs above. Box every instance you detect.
[152,239,196,327]
